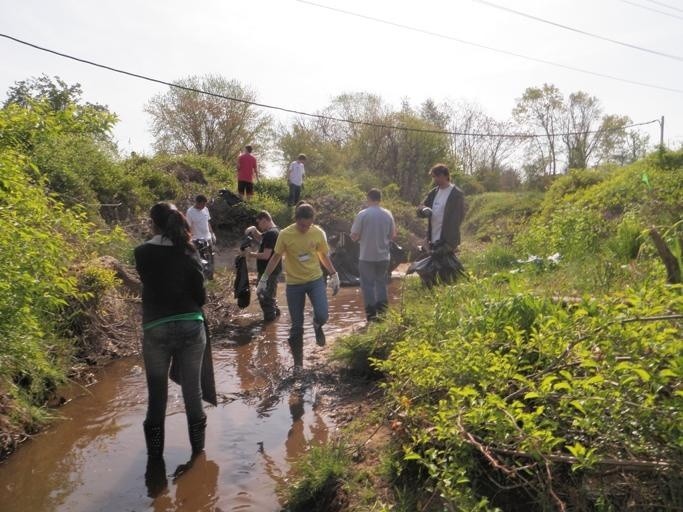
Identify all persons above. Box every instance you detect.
[261,363,330,512]
[144,451,220,511]
[285,152,307,208]
[235,144,260,201]
[185,193,215,281]
[254,198,342,365]
[415,162,466,263]
[348,185,398,324]
[132,200,217,461]
[256,317,278,414]
[238,209,281,323]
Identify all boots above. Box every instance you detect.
[312,318,327,346]
[287,338,304,376]
[143,420,168,488]
[356,301,388,335]
[188,413,207,453]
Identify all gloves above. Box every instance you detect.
[245,226,256,235]
[422,207,433,216]
[256,279,268,301]
[330,272,341,296]
